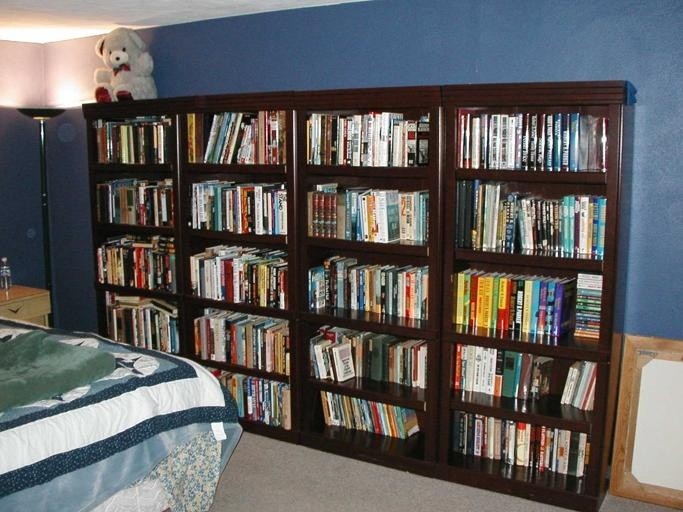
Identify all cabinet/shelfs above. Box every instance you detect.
[439,76,636,512]
[80,94,186,358]
[179,89,300,445]
[298,83,439,481]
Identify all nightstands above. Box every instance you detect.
[0,284,51,326]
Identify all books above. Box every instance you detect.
[306,110,431,439]
[95,114,179,358]
[189,110,292,431]
[451,107,605,477]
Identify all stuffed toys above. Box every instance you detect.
[93,21,160,105]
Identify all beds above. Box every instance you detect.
[0,314,245,512]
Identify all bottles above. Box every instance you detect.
[0,257,12,288]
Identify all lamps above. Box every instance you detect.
[17,108,66,329]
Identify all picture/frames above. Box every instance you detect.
[609,327,683,510]
